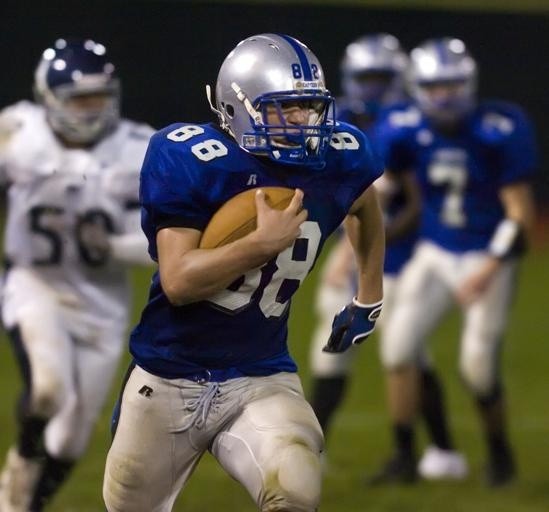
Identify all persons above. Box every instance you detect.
[334,32,540,486]
[102,26,388,512]
[0,31,162,512]
[308,28,467,482]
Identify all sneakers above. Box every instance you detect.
[370,443,516,488]
[0,445,46,512]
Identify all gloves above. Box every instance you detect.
[323,296,383,353]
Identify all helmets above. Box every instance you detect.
[206,33,336,169]
[340,34,410,115]
[34,38,121,145]
[406,39,479,120]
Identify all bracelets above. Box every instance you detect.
[487,215,532,262]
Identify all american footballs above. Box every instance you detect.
[199,186,303,250]
